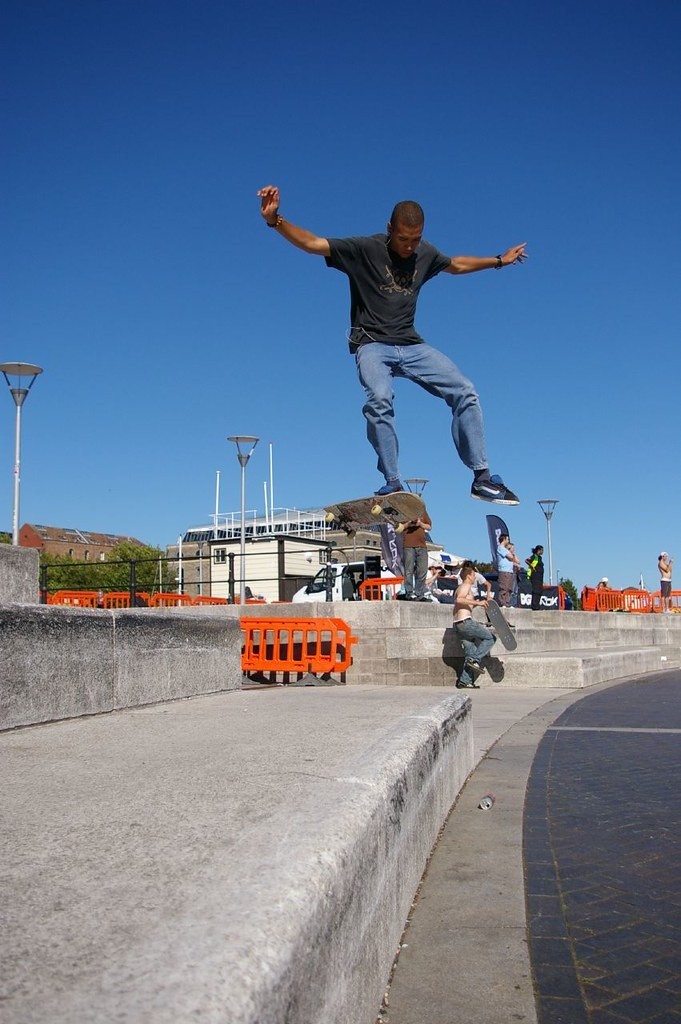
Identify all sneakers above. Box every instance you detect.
[471,476,520,505]
[377,482,405,497]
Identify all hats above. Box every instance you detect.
[661,551,668,557]
[602,578,608,582]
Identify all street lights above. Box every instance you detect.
[227,435,260,605]
[0,362,45,546]
[536,499,559,586]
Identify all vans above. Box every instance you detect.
[292,557,439,603]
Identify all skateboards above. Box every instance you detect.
[484,599,517,652]
[323,491,428,538]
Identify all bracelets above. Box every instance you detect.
[266,213,283,228]
[494,254,503,269]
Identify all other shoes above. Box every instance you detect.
[457,681,479,689]
[533,606,547,610]
[406,596,419,600]
[415,596,432,601]
[664,609,670,613]
[466,659,485,674]
[502,605,506,608]
[509,606,514,608]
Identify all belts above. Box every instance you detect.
[453,617,472,625]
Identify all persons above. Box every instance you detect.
[597,576,613,591]
[496,533,520,609]
[525,545,545,611]
[453,566,497,689]
[422,565,457,603]
[257,180,527,507]
[458,560,491,605]
[658,551,674,613]
[398,510,434,603]
